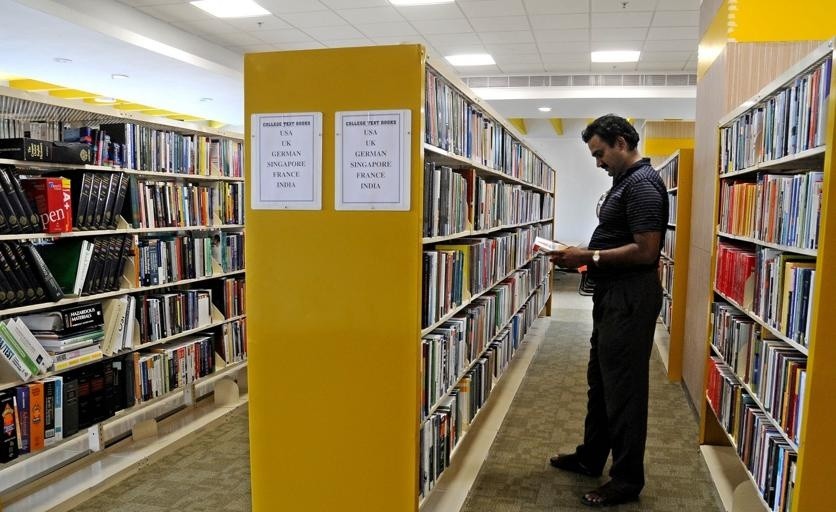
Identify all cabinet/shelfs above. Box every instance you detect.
[243,45,558,512]
[654,148,698,380]
[701,42,836,512]
[0,85,246,510]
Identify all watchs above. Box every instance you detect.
[592,249,600,267]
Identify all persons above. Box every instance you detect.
[539,113,669,508]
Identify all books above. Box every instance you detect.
[423,158,554,329]
[0,163,245,235]
[717,171,823,249]
[705,300,808,512]
[718,61,827,174]
[0,121,243,177]
[425,70,553,194]
[714,239,817,351]
[0,228,245,311]
[533,235,585,260]
[419,312,524,499]
[0,274,246,463]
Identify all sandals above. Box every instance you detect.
[549,452,603,477]
[581,486,638,506]
